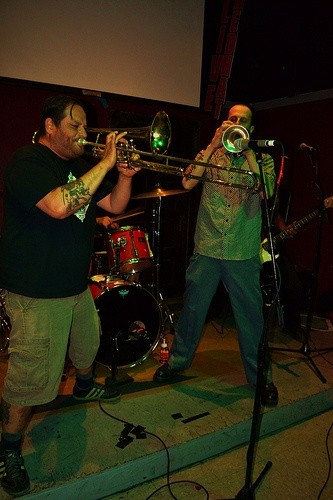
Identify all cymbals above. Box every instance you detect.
[129,188,190,199]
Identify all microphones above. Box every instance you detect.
[233,138,280,150]
[300,143,316,151]
[138,330,150,341]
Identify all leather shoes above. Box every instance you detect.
[153,363,184,383]
[252,382,278,407]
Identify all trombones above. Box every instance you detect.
[32,111,262,192]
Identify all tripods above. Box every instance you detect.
[268,150,333,387]
[236,149,273,500]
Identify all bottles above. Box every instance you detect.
[160,338,169,364]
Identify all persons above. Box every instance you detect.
[152,102,279,407]
[95,215,120,231]
[0,96,142,497]
[261,193,307,344]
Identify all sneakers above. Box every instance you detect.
[73,381,121,402]
[0,443,30,497]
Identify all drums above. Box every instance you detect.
[106,226,155,275]
[88,273,163,372]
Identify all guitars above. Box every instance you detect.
[259,193,333,264]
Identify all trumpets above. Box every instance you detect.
[222,124,250,154]
